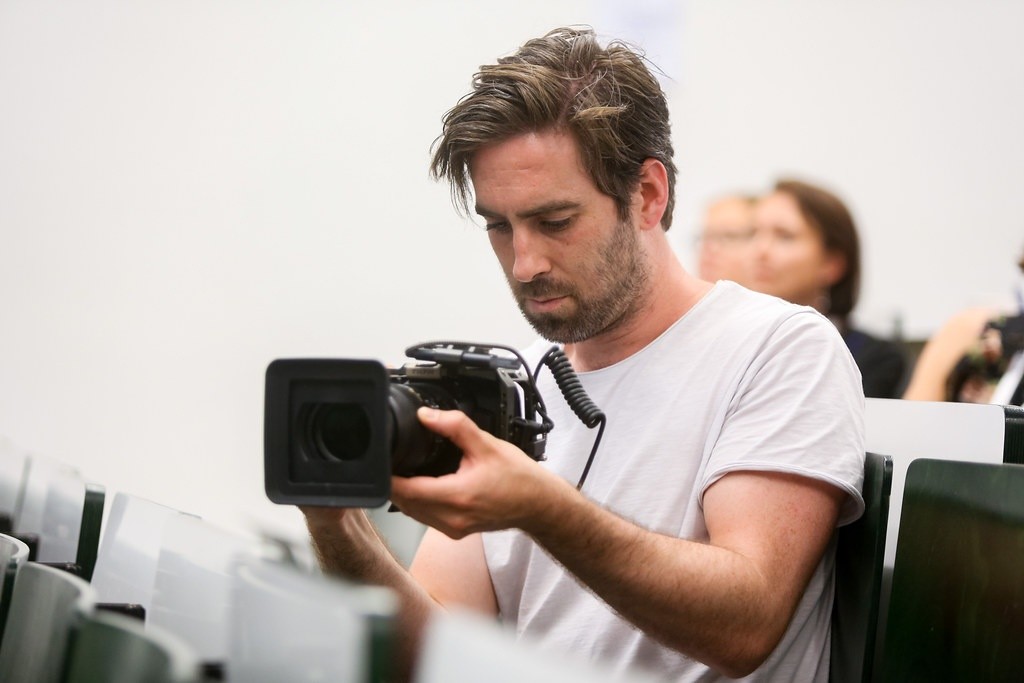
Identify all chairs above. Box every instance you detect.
[2,339,1024,683]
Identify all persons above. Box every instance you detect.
[699,181,1024,401]
[294,33,866,683]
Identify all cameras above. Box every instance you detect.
[265,343,553,509]
